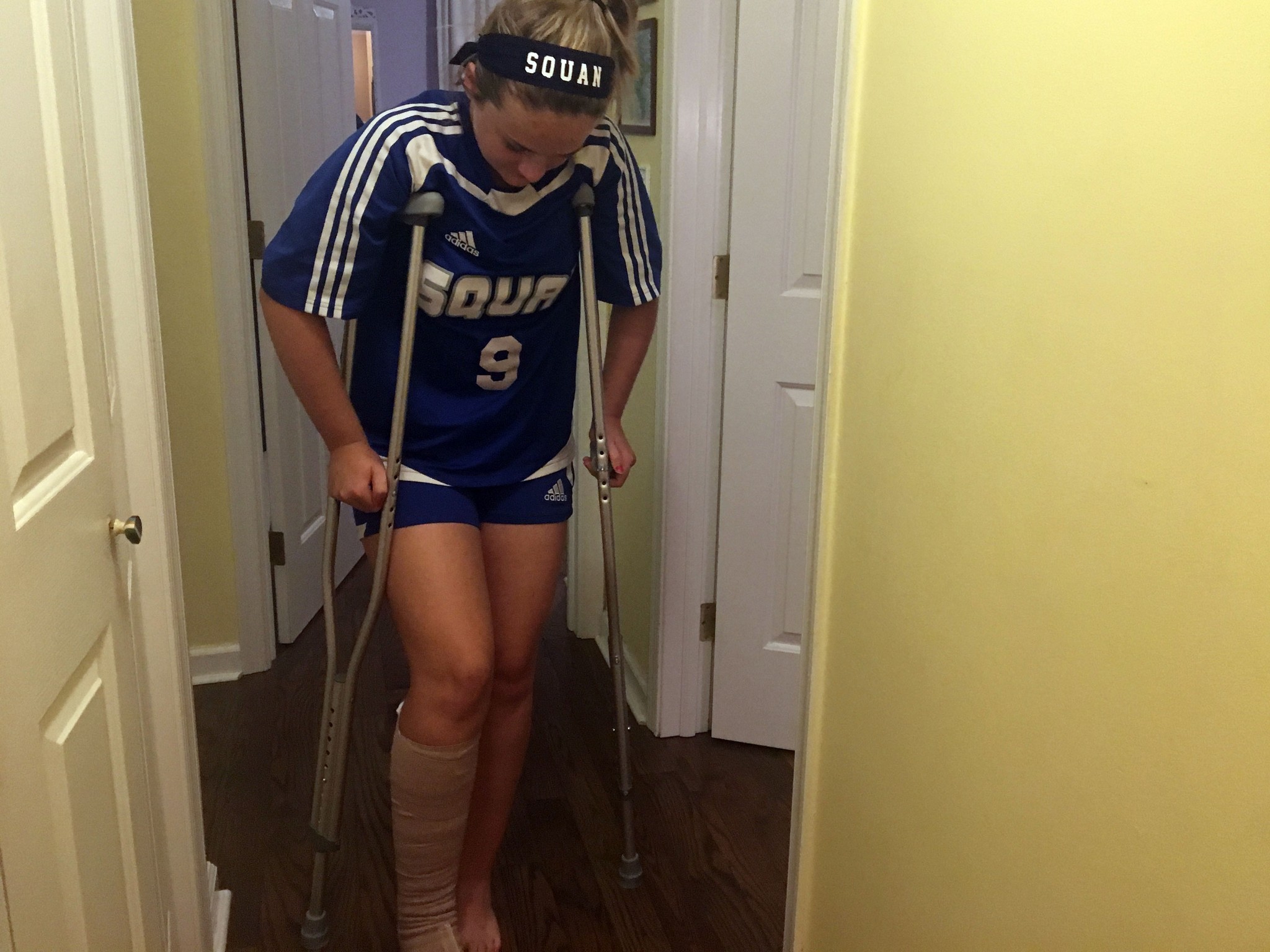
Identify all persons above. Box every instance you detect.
[259,1,663,952]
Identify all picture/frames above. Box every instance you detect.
[617,17,658,136]
